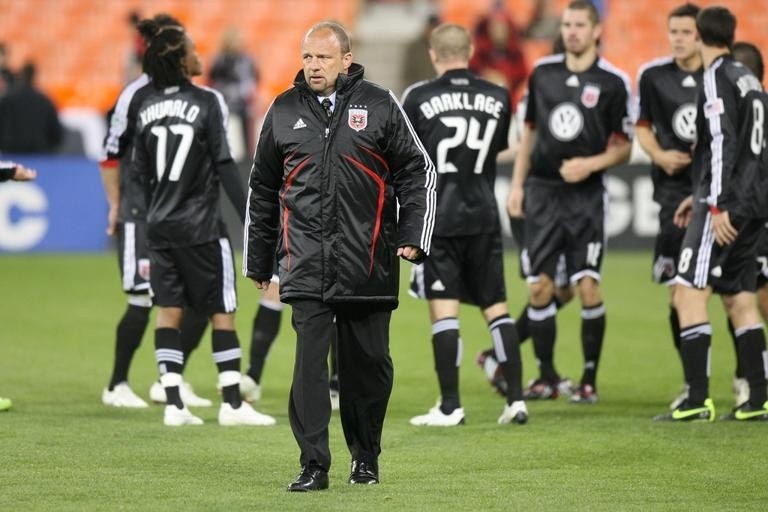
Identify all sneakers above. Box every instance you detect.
[0,397,11,410]
[497,401,528,425]
[478,352,599,403]
[102,375,276,425]
[409,404,465,427]
[329,391,340,412]
[653,375,768,421]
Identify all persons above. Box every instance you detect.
[401,0,564,114]
[636,3,768,423]
[219,275,339,411]
[404,23,534,428]
[479,2,633,406]
[242,21,438,490]
[0,61,66,182]
[100,12,278,425]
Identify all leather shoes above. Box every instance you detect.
[349,459,378,485]
[287,466,329,491]
